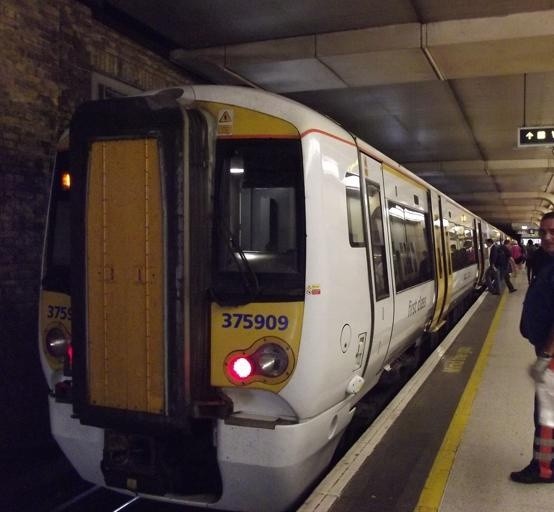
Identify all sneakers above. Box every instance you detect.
[511,460,554,483]
[509,287,517,293]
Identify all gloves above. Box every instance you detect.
[527,353,551,383]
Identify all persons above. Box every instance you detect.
[510,212,554,483]
[487,238,539,296]
[418,241,476,283]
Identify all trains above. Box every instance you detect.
[37,84,518,512]
